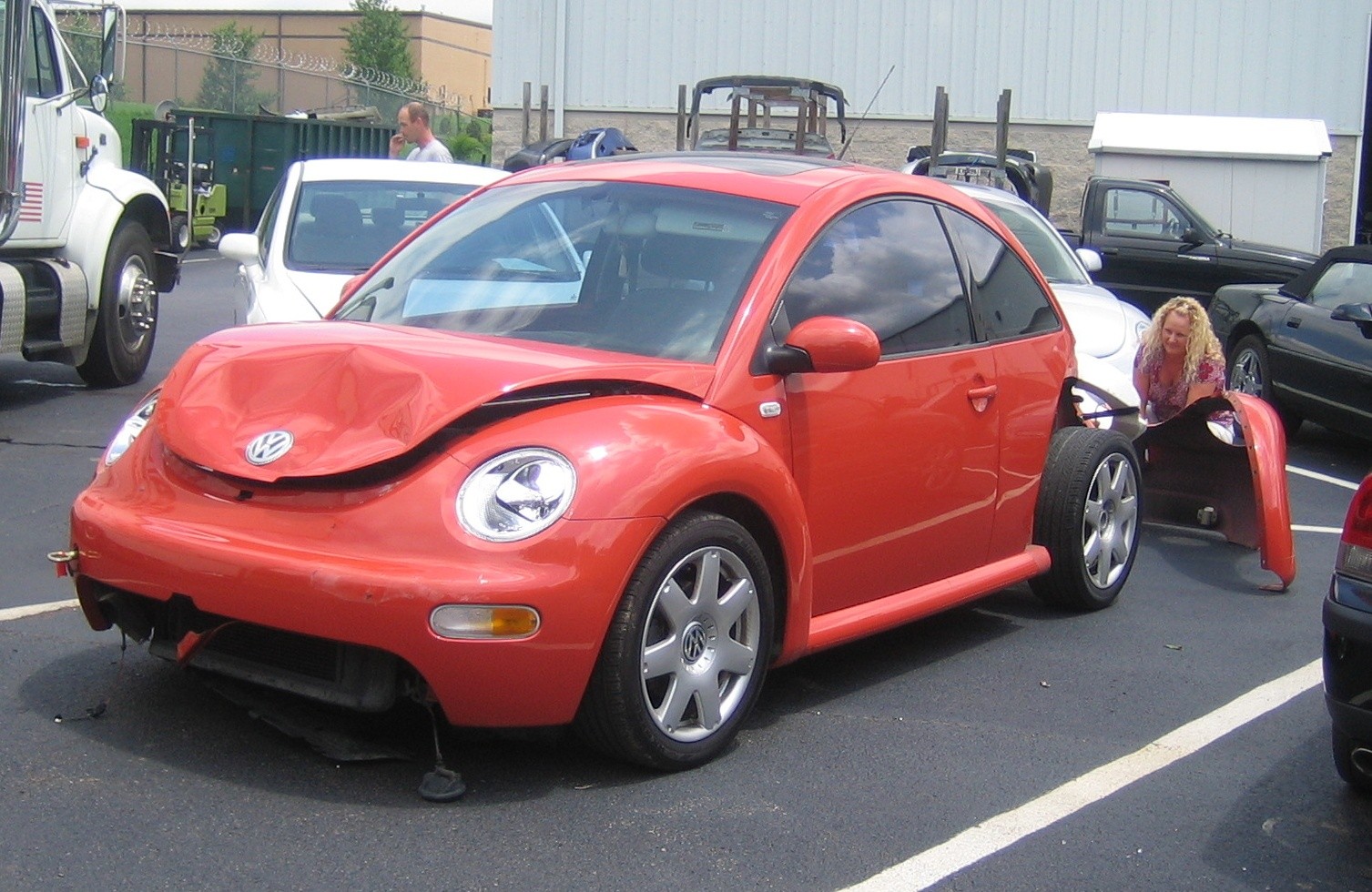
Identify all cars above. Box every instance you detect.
[212,158,598,340]
[1203,244,1372,446]
[45,150,1144,775]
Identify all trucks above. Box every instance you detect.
[0,0,199,394]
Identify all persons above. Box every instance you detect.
[1132,295,1234,428]
[386,101,455,162]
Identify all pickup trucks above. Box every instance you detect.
[1015,177,1344,318]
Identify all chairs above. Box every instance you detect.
[371,207,405,225]
[309,194,345,223]
[426,202,450,218]
[605,236,722,349]
[297,199,380,258]
